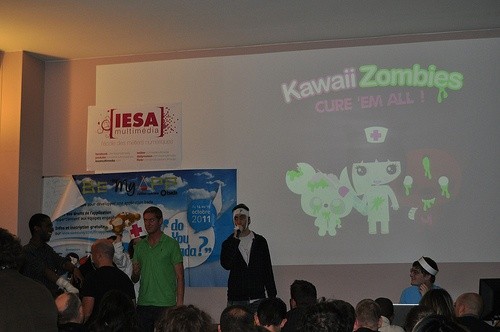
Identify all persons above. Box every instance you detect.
[0,213,500,332]
[131,207,184,321]
[220,204,277,309]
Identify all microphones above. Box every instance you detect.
[236,229,241,238]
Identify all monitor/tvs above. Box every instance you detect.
[390,304,420,327]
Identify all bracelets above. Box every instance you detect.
[133,272,138,278]
[116,233,122,236]
